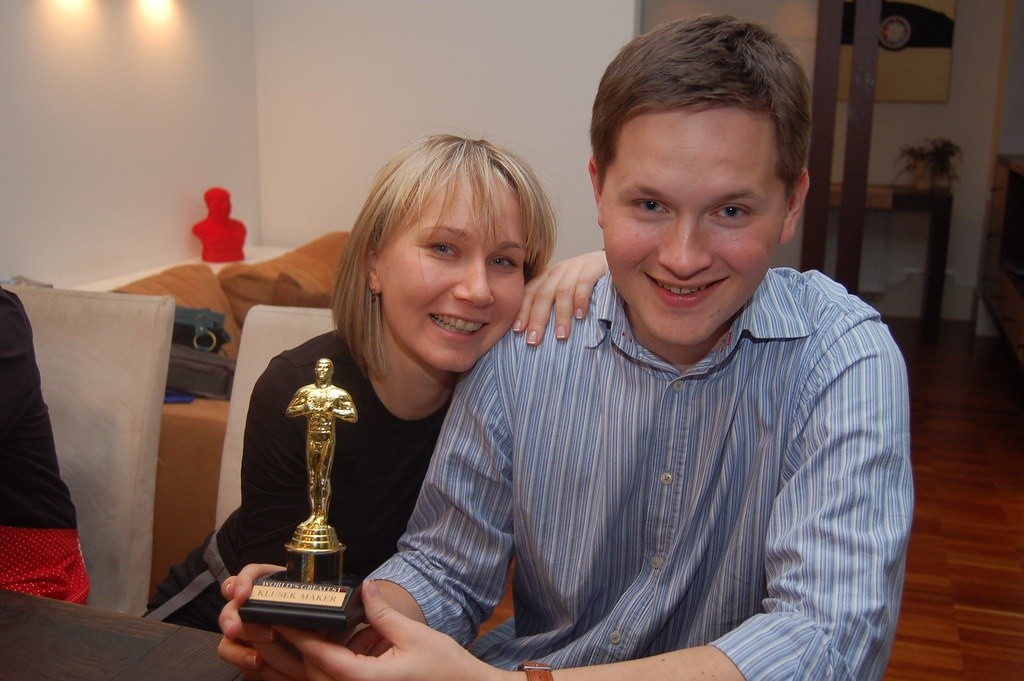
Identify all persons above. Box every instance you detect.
[216,15,912,681]
[138,135,608,633]
[0,287,92,602]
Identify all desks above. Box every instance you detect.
[0,588,250,681]
[827,184,955,345]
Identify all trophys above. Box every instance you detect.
[239,357,367,627]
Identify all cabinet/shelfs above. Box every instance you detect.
[981,154,1024,364]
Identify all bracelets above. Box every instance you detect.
[520,662,554,681]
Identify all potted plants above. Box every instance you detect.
[888,137,960,191]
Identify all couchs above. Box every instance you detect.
[108,231,357,612]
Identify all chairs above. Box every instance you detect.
[214,304,338,533]
[0,286,176,621]
